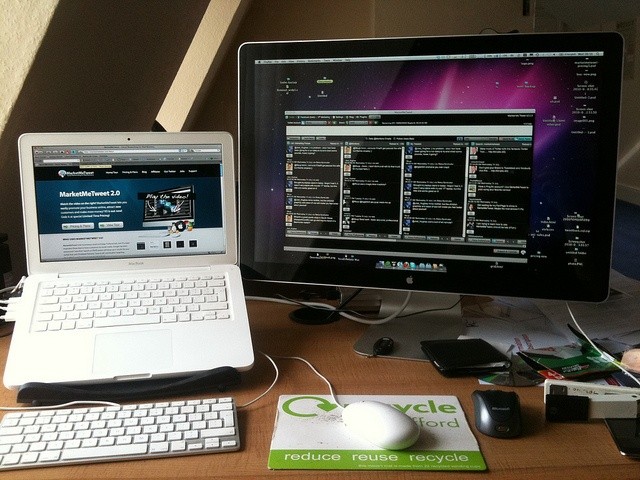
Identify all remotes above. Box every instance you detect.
[544,379,640,404]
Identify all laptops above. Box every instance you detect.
[3,132,255,391]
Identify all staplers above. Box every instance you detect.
[543,376,640,419]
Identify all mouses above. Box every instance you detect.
[342,401,420,450]
[471,390,523,438]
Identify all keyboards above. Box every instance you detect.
[0,395,241,471]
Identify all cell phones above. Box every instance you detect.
[603,418,640,459]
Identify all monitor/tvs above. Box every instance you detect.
[238,32,625,303]
